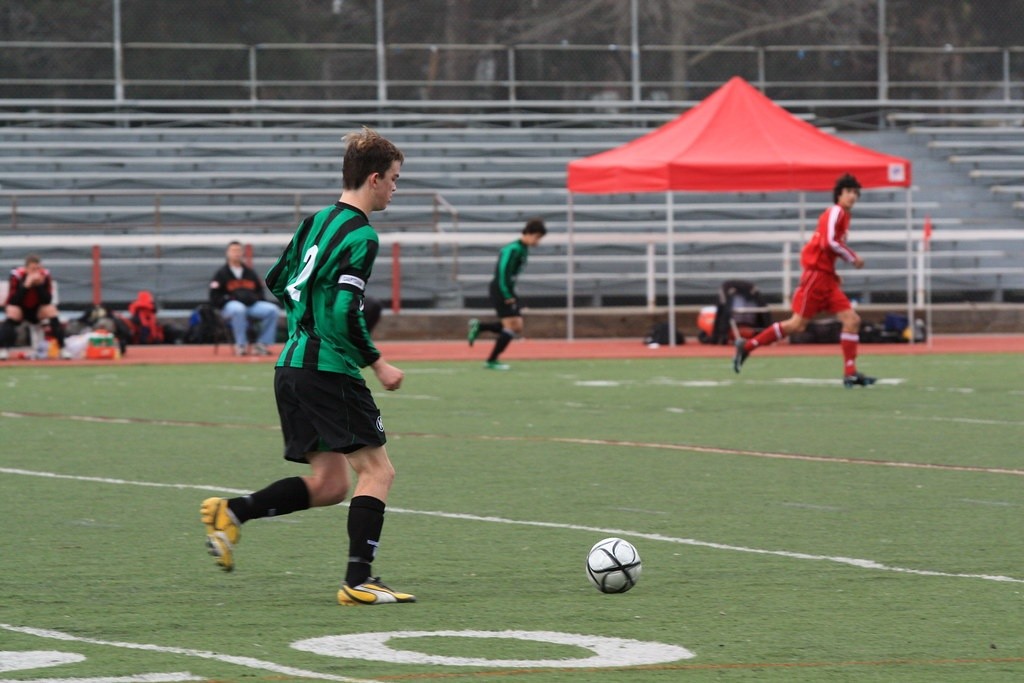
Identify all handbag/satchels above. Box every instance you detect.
[184,305,229,345]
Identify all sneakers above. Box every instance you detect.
[843,372,876,388]
[337,576,416,605]
[200,496,242,571]
[467,318,479,345]
[484,361,509,370]
[733,338,748,372]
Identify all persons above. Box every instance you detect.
[730,174,878,388]
[199,124,416,606]
[466,220,548,370]
[208,240,279,356]
[0,255,72,360]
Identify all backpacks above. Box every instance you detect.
[642,322,685,347]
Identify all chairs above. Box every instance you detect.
[207,301,263,357]
[719,281,775,347]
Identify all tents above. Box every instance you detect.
[567,75,916,346]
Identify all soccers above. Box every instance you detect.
[584,537,644,596]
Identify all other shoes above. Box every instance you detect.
[252,343,272,355]
[235,344,248,354]
